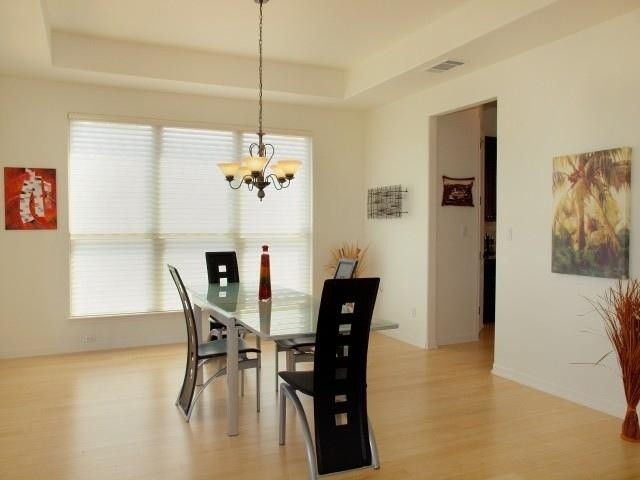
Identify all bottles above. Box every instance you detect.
[258,244,272,302]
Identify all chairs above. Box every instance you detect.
[204,251,262,371]
[276,273,379,480]
[167,263,263,423]
[273,259,358,393]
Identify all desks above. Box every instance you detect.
[184,284,399,438]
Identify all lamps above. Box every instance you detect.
[217,1,301,200]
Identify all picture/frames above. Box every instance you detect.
[440,175,475,207]
